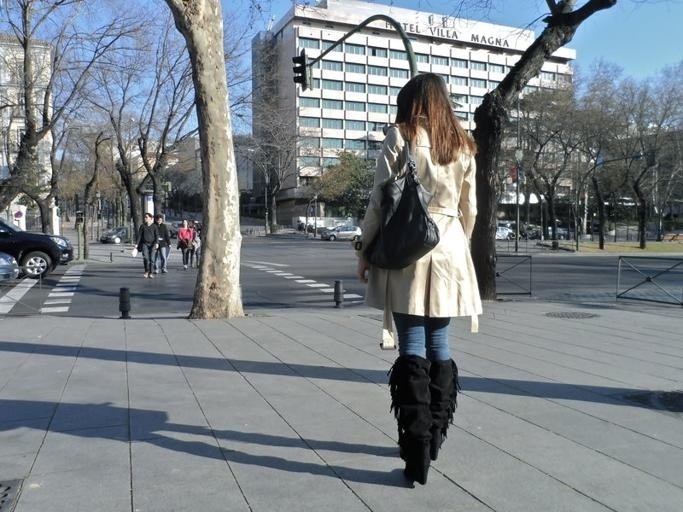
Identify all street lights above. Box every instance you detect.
[245,148,269,211]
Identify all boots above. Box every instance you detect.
[387,353,459,487]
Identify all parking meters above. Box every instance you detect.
[76,210,83,262]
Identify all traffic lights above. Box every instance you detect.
[292,47,307,92]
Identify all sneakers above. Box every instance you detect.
[141,262,201,280]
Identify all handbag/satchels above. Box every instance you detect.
[360,129,442,272]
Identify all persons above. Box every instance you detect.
[354,72,483,485]
[520,221,528,240]
[135,212,202,279]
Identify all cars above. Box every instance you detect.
[321,225,361,243]
[0,252,18,286]
[100,225,136,245]
[193,223,202,237]
[495,216,599,242]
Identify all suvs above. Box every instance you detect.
[1,217,74,279]
[169,221,182,237]
